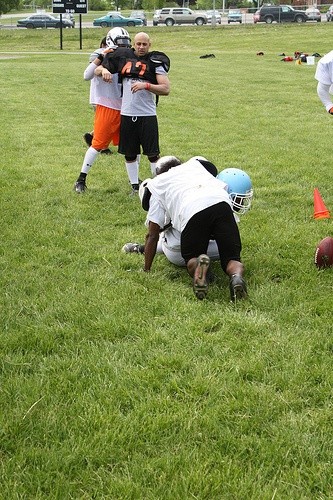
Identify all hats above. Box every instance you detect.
[139,178,152,211]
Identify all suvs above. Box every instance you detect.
[258,3,309,24]
[159,7,209,26]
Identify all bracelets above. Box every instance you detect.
[145,83,150,91]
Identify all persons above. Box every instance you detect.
[73,26,147,192]
[137,155,250,304]
[121,167,255,267]
[94,31,170,193]
[314,50,333,115]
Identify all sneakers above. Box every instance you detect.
[74,179,86,193]
[130,189,139,196]
[127,178,143,184]
[229,277,249,302]
[193,254,210,300]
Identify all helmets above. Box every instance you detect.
[216,167,251,198]
[156,156,180,175]
[105,27,131,48]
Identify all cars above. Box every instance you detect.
[227,9,243,23]
[93,12,143,28]
[128,11,148,26]
[305,8,322,22]
[253,7,264,24]
[206,10,222,24]
[325,5,333,22]
[17,14,74,29]
[153,10,163,26]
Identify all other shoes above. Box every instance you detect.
[83,132,112,154]
